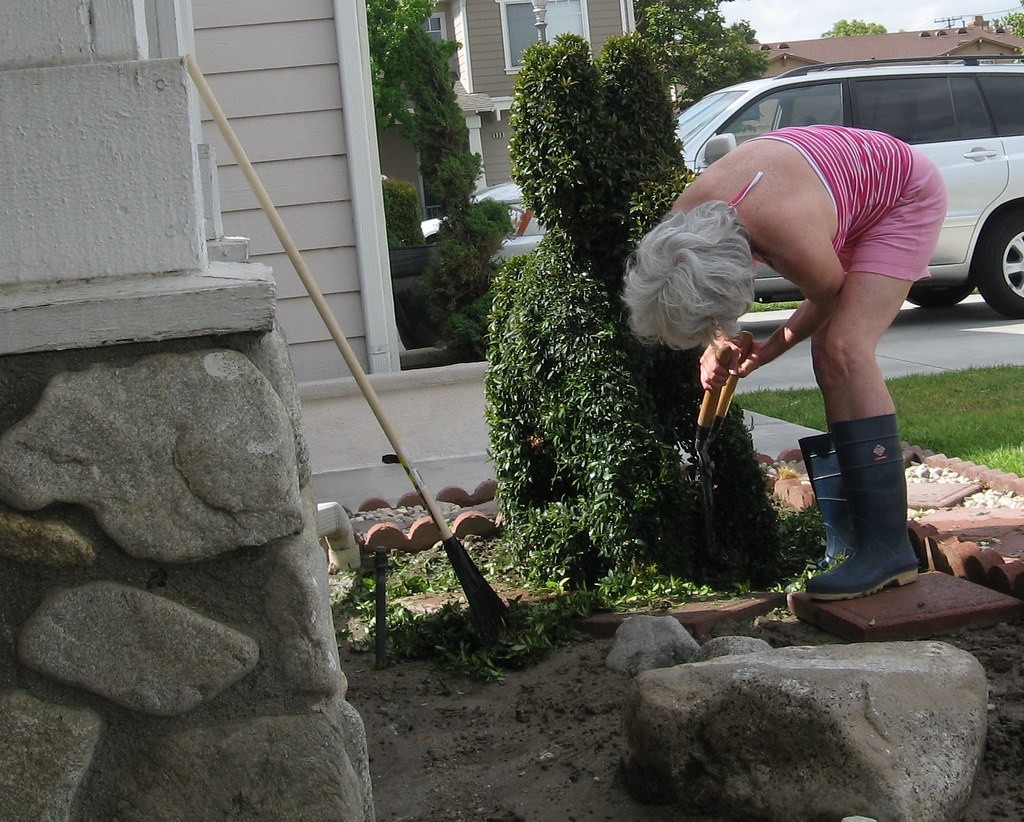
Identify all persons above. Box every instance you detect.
[618,123,948,602]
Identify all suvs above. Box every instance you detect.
[469,52,1024,320]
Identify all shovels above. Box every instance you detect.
[187,58,508,642]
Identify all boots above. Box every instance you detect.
[798,433,858,570]
[806,415,918,600]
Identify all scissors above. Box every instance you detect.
[689,330,753,568]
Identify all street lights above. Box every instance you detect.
[531,0,548,43]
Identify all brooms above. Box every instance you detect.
[184,52,511,644]
